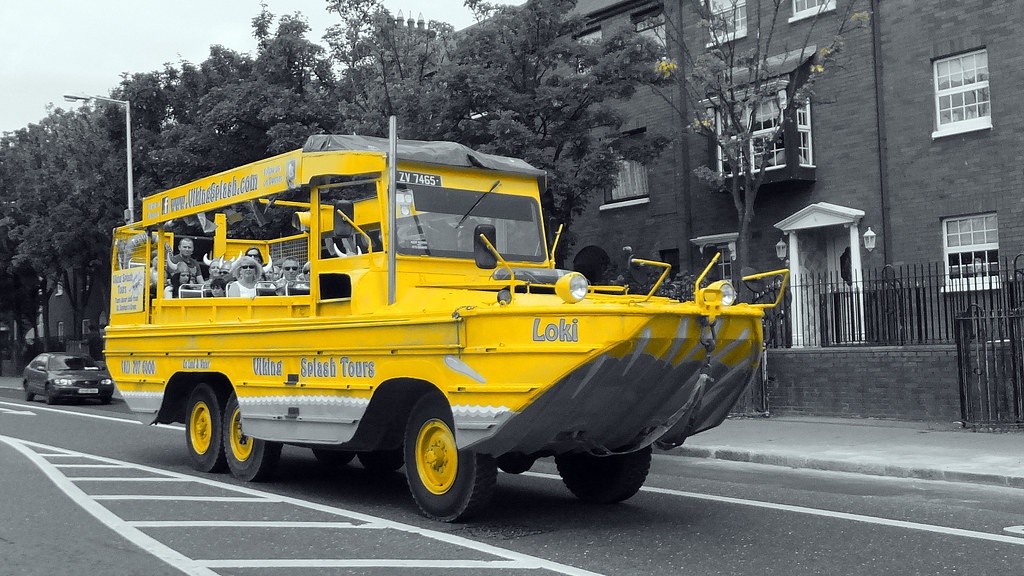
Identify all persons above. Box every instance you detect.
[302,237,351,299]
[259,257,310,295]
[244,247,263,265]
[150,250,167,286]
[165,263,191,298]
[166,238,205,285]
[228,256,262,297]
[208,258,236,297]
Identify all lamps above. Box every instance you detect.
[775,238,788,261]
[863,227,876,252]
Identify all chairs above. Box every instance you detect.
[164,280,310,299]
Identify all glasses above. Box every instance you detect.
[241,265,255,269]
[219,271,229,275]
[304,269,310,274]
[284,267,298,271]
[247,254,259,258]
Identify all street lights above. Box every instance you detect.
[63,92,135,226]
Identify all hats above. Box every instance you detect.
[167,251,197,274]
[203,253,235,266]
[219,251,243,271]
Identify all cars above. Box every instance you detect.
[22,352,114,405]
